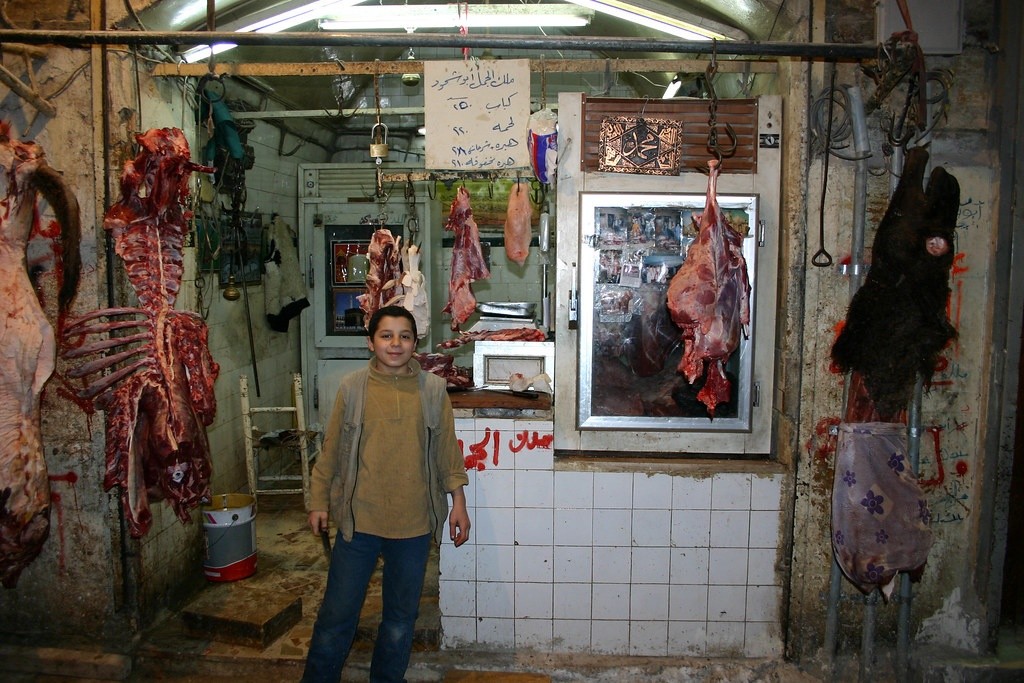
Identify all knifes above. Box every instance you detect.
[491,389,539,401]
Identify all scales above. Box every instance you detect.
[461,211,551,339]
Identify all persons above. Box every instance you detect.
[302,306,471,683]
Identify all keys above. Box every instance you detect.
[375,157,383,165]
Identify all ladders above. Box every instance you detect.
[238,371,313,515]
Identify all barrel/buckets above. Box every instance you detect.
[199,492,259,582]
